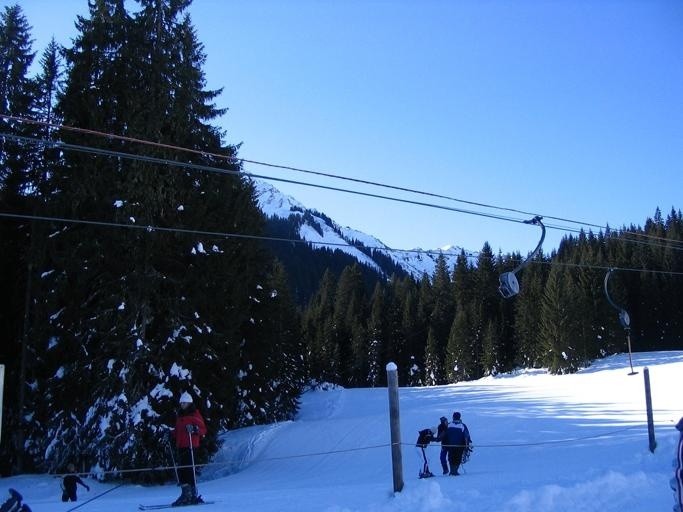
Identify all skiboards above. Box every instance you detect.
[139,499,221,511]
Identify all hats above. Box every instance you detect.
[179,392,193,402]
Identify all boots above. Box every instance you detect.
[172,483,196,505]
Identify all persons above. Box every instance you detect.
[60,464,90,501]
[415,426,437,479]
[162,391,207,506]
[437,417,451,474]
[442,412,471,476]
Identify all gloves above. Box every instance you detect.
[186,425,193,433]
